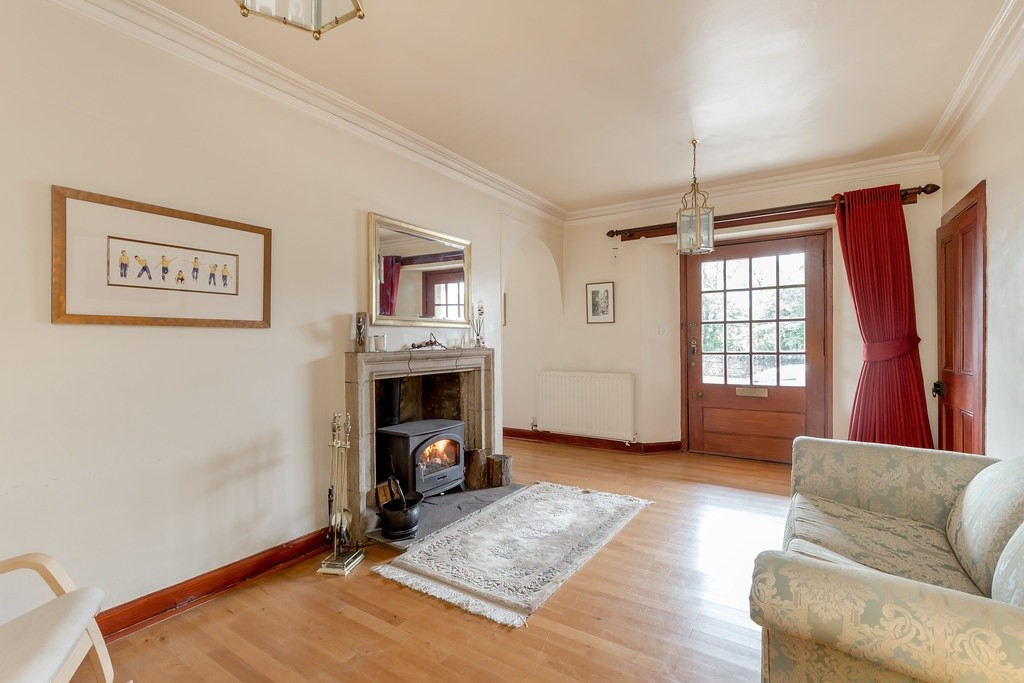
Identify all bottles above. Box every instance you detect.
[374,334,386,352]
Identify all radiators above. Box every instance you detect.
[531,370,637,447]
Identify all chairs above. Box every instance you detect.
[0,552,134,683]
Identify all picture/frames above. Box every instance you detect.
[586,281,615,323]
[51,184,272,329]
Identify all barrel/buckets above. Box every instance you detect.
[378,476,420,540]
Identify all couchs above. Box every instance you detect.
[749,435,1024,683]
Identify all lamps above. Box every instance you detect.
[235,0,366,40]
[676,140,715,257]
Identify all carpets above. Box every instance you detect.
[372,480,655,630]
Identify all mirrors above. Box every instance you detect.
[369,212,473,328]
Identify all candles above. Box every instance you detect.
[375,337,384,350]
